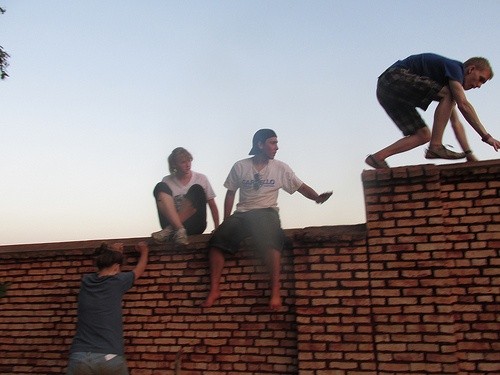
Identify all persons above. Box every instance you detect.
[200,128,333,309]
[365,53,500,169]
[152,147,220,245]
[66,242,148,375]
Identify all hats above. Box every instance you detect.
[249,129,277,155]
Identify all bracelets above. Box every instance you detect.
[481,133,491,142]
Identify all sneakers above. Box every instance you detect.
[425,146,466,159]
[152,228,174,244]
[365,155,389,168]
[174,229,189,247]
